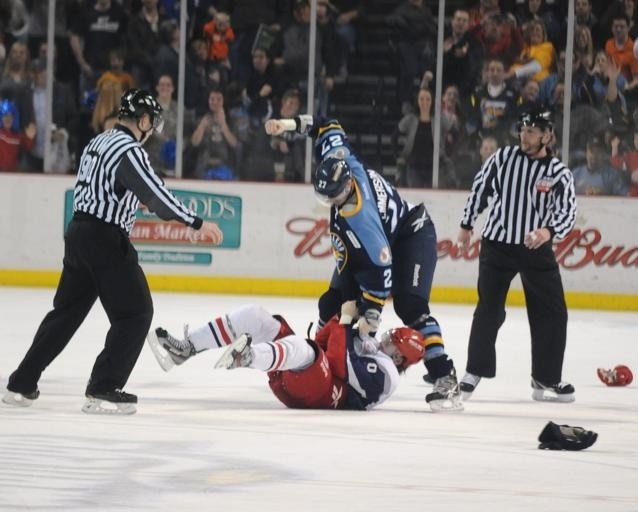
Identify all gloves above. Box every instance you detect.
[597,365,633,386]
[538,422,597,450]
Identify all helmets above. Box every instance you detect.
[0,100,20,131]
[517,107,553,129]
[382,328,425,367]
[119,88,164,133]
[314,157,351,203]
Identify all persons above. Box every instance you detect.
[264,113,461,412]
[458,107,577,403]
[148,305,426,409]
[1,0,637,197]
[7,88,222,413]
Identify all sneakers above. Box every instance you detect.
[7,384,39,399]
[85,387,138,402]
[531,377,575,394]
[459,371,481,392]
[227,332,253,370]
[156,327,196,365]
[426,375,459,403]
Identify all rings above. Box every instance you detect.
[529,231,534,236]
[531,235,537,239]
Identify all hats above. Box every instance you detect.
[586,136,611,155]
